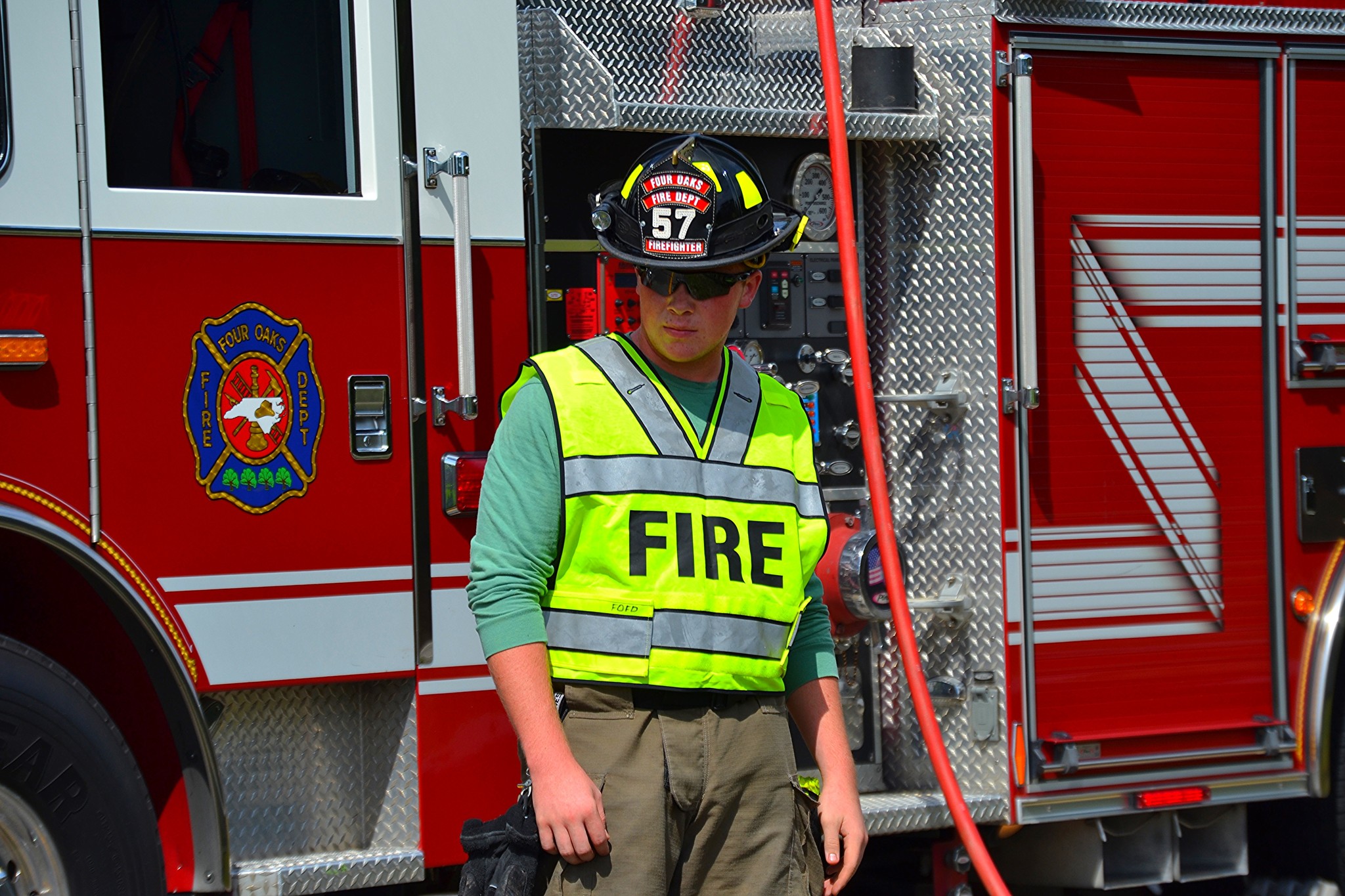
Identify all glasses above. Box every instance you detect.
[633,264,753,301]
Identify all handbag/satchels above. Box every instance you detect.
[459,793,538,896]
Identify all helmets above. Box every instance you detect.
[587,134,809,269]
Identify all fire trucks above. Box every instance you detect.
[3,0,1343,895]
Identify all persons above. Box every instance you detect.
[465,133,870,895]
